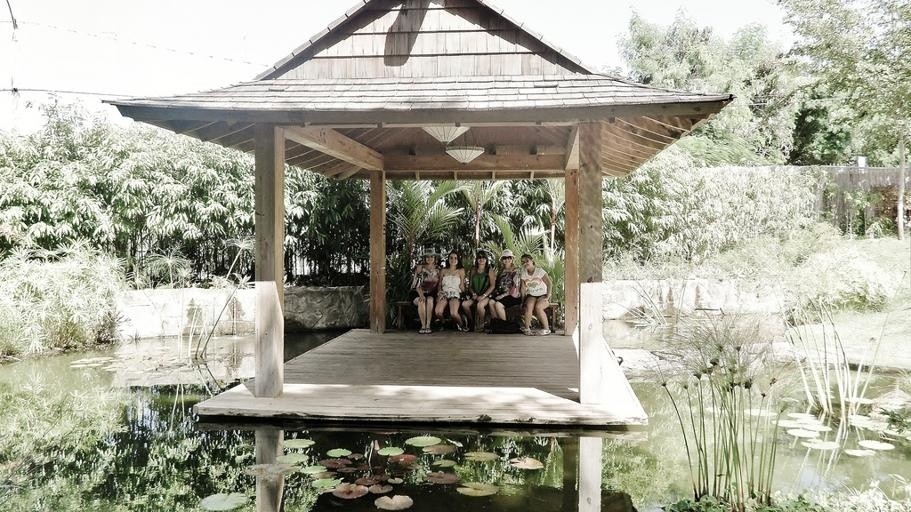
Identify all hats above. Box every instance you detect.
[420,249,440,257]
[499,250,514,263]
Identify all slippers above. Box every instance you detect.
[418,327,432,334]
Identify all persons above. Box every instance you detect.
[434,248,467,331]
[488,249,522,321]
[409,248,440,335]
[520,254,553,336]
[462,249,497,334]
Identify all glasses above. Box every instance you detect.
[479,256,484,259]
[501,256,512,260]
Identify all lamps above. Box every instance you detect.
[420,125,485,165]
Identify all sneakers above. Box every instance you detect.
[456,316,485,332]
[524,327,551,336]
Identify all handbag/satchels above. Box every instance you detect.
[490,317,520,334]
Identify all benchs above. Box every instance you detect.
[397,301,560,334]
